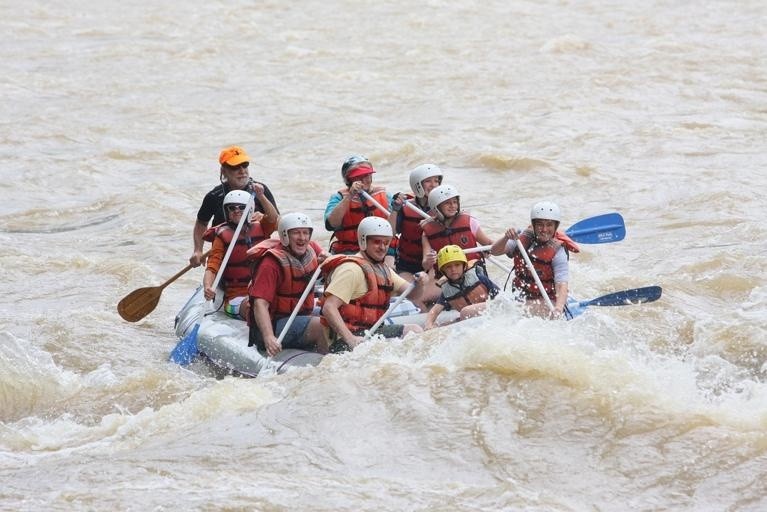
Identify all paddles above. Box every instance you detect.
[462,210,627,255]
[567,284,670,309]
[117,249,214,323]
[167,185,258,367]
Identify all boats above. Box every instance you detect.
[171,248,594,384]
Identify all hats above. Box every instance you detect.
[219,146,251,167]
[346,167,376,178]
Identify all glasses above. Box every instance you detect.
[222,161,249,171]
[226,204,246,212]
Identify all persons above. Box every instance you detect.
[190,146,280,268]
[319,216,423,353]
[247,213,332,357]
[409,185,495,312]
[425,244,498,331]
[490,200,580,320]
[202,183,280,318]
[324,155,399,271]
[389,165,444,283]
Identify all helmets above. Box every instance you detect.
[409,164,444,199]
[530,200,563,239]
[341,154,376,184]
[357,215,394,252]
[222,189,255,224]
[277,213,313,247]
[437,244,468,271]
[427,184,461,221]
